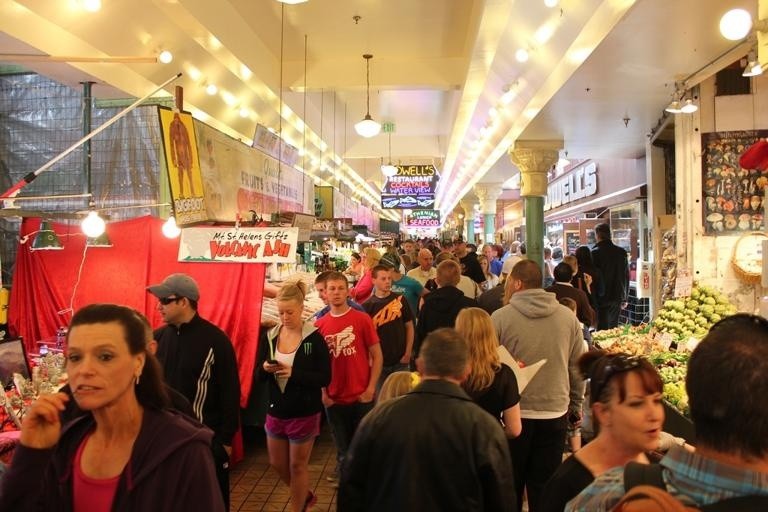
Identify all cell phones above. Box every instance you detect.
[267,360,279,367]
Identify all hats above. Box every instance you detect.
[453,234,468,244]
[146,273,199,301]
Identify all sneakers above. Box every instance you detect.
[326,472,337,481]
[302,490,317,511]
[331,479,338,488]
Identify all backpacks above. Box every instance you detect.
[604,463,768,511]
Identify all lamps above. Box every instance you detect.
[379,122,399,178]
[353,54,382,139]
[665,90,682,113]
[30,215,64,252]
[80,196,105,238]
[83,234,111,246]
[161,205,184,242]
[682,92,696,114]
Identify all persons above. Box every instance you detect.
[565,314,767,512]
[346,248,381,303]
[406,249,439,286]
[264,283,284,298]
[544,249,552,287]
[478,244,502,277]
[401,239,415,264]
[418,252,482,309]
[493,244,503,257]
[417,237,450,249]
[558,298,592,453]
[502,242,526,282]
[450,235,487,284]
[337,329,517,511]
[386,247,405,273]
[341,252,362,280]
[538,349,665,509]
[545,262,596,320]
[377,371,420,402]
[253,280,331,511]
[314,275,383,487]
[589,223,630,329]
[552,255,592,294]
[167,112,196,198]
[369,251,430,329]
[575,246,605,327]
[362,266,414,374]
[2,304,226,511]
[398,254,410,266]
[488,260,587,511]
[146,274,241,511]
[410,260,483,360]
[314,272,364,318]
[453,308,523,439]
[477,257,526,314]
[502,241,520,258]
[553,247,563,267]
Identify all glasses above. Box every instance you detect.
[593,355,639,402]
[159,296,182,305]
